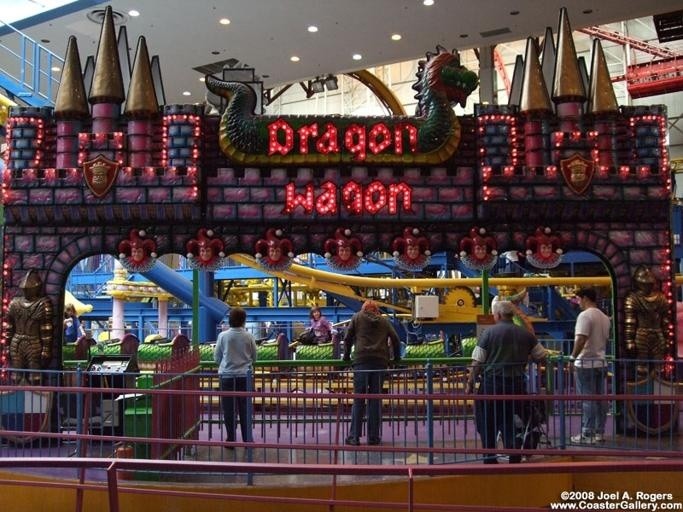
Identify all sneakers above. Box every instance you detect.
[344,434,360,445]
[366,437,381,444]
[593,432,603,442]
[570,433,595,444]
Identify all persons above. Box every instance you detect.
[297,308,337,346]
[343,301,403,445]
[213,307,257,448]
[463,300,548,464]
[569,286,612,445]
